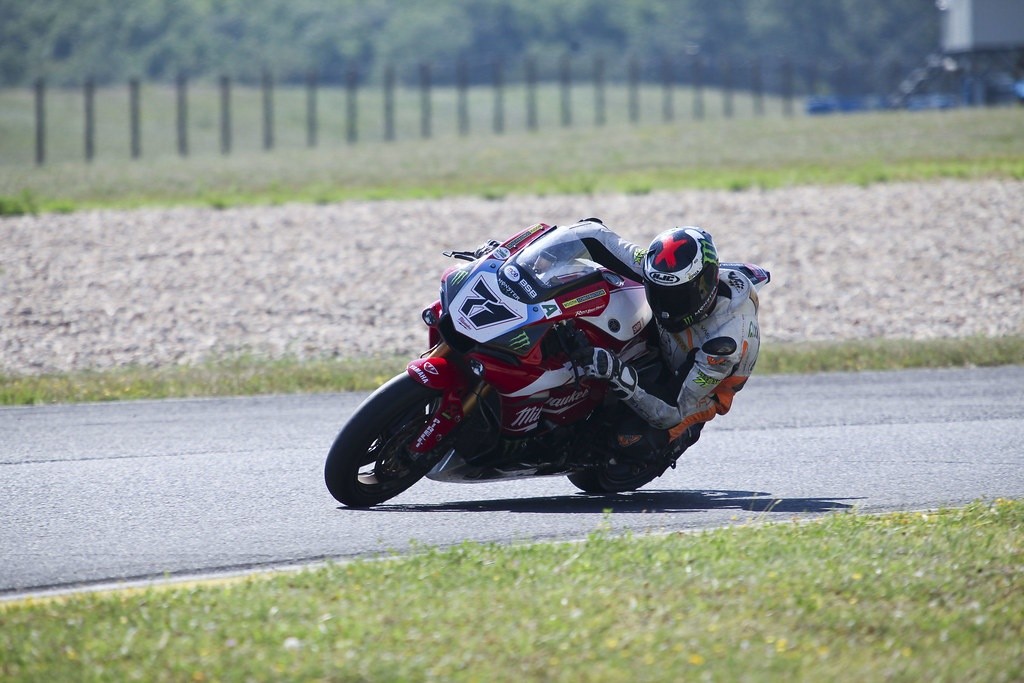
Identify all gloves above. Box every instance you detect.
[571,347,638,401]
[476,240,501,260]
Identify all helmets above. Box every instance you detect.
[642,226,718,333]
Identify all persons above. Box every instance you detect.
[533,220,760,470]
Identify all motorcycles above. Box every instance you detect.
[325,221,772,508]
